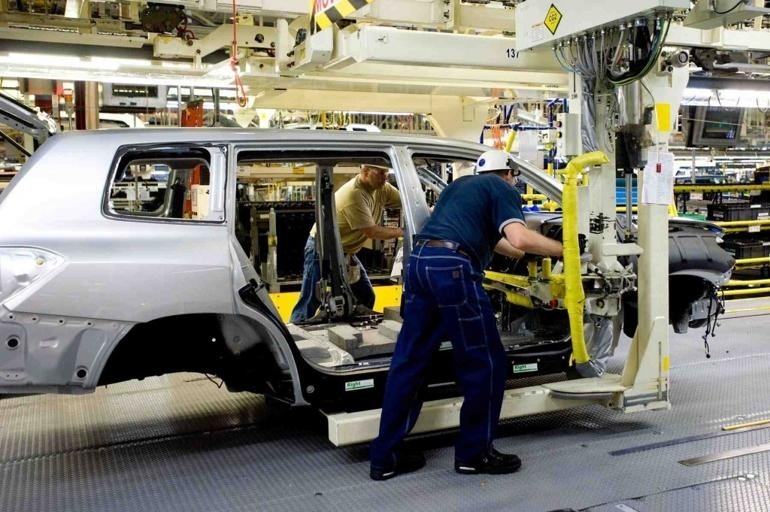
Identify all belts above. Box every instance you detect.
[415,239,469,256]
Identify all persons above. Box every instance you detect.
[289,163,404,325]
[369,149,588,481]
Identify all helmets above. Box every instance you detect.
[475,150,516,173]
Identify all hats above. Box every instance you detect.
[360,164,389,170]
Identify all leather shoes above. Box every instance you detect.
[455,448,522,474]
[370,453,427,480]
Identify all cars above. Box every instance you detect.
[0,86,741,415]
